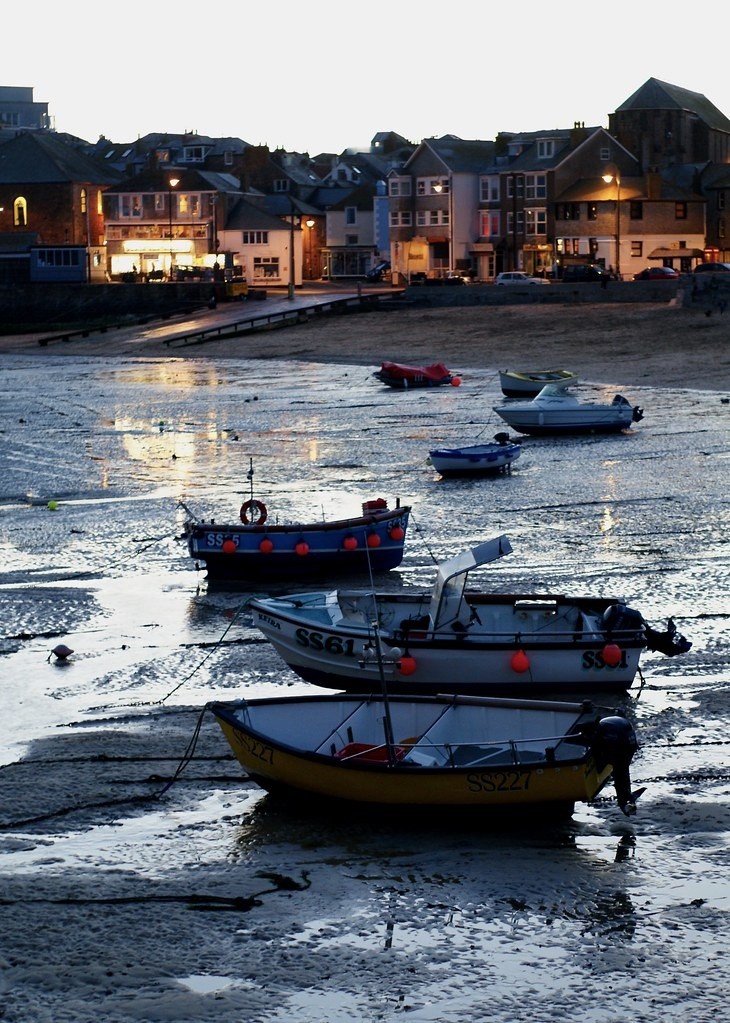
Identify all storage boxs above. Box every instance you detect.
[334,742,405,763]
[361,500,388,514]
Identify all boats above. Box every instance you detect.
[493,384,645,437]
[497,369,579,398]
[185,497,413,583]
[373,360,452,390]
[205,694,648,817]
[247,534,694,702]
[429,432,522,481]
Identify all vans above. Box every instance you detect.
[563,264,613,282]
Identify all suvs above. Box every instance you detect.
[495,271,551,287]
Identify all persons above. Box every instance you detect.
[104,271,111,282]
[607,265,613,273]
[133,264,137,272]
[468,268,474,284]
[541,267,547,279]
[152,263,155,272]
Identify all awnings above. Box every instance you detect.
[647,248,702,258]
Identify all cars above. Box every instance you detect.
[694,261,730,272]
[633,266,679,281]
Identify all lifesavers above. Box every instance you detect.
[240,500,267,525]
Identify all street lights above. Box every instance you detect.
[168,178,180,281]
[601,163,621,282]
[305,217,316,280]
